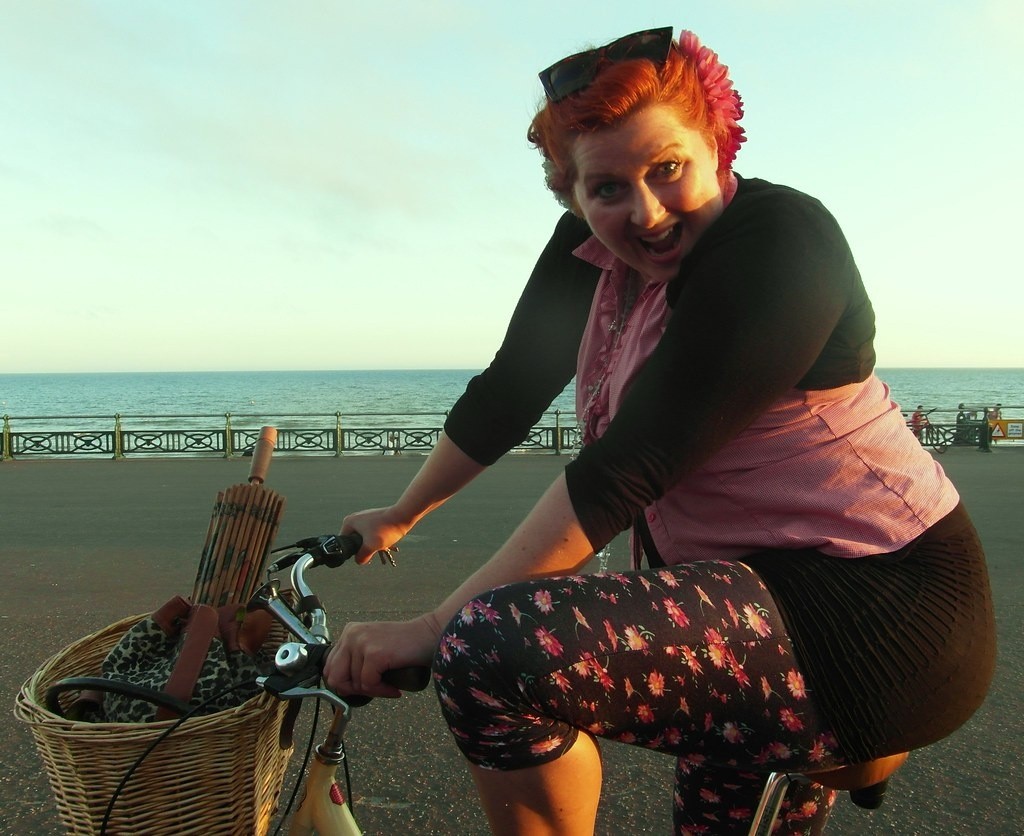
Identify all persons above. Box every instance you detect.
[989,404,1001,444]
[956,403,966,424]
[967,411,977,419]
[321,27,999,836]
[912,405,924,445]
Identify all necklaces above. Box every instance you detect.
[568,270,635,464]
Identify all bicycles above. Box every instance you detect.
[9,526,911,836]
[901,407,947,454]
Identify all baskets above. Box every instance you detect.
[14,611,293,836]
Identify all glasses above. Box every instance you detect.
[539,26,674,101]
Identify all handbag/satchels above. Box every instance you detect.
[63,596,272,719]
